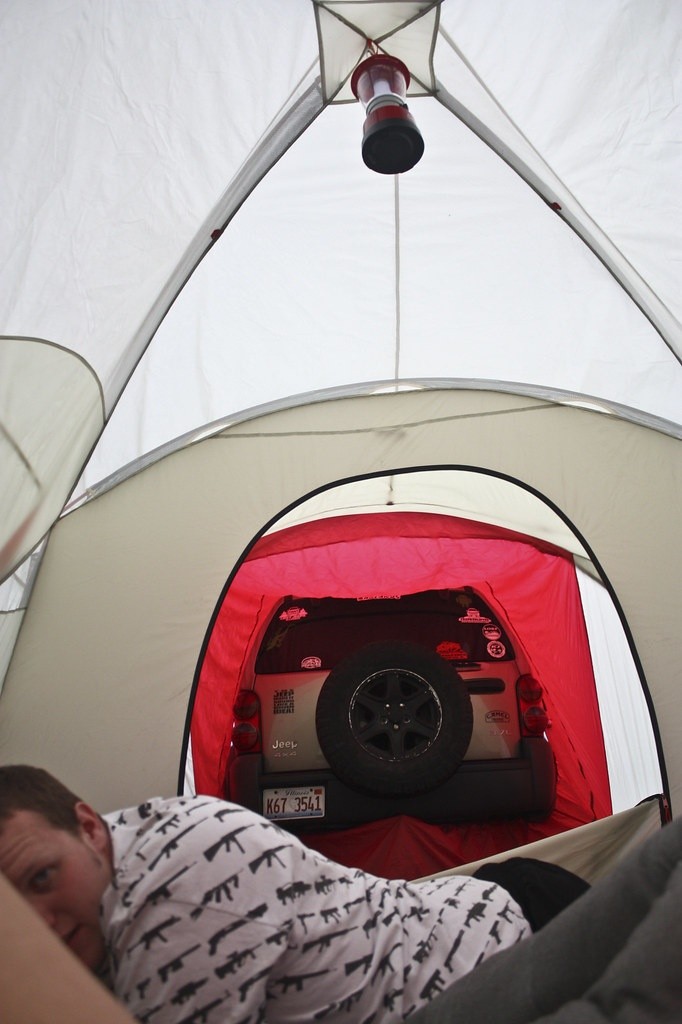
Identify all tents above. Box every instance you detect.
[185,507,615,884]
[4,0,681,853]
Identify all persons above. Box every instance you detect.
[1,762,598,1024]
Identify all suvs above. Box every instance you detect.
[226,587,557,837]
